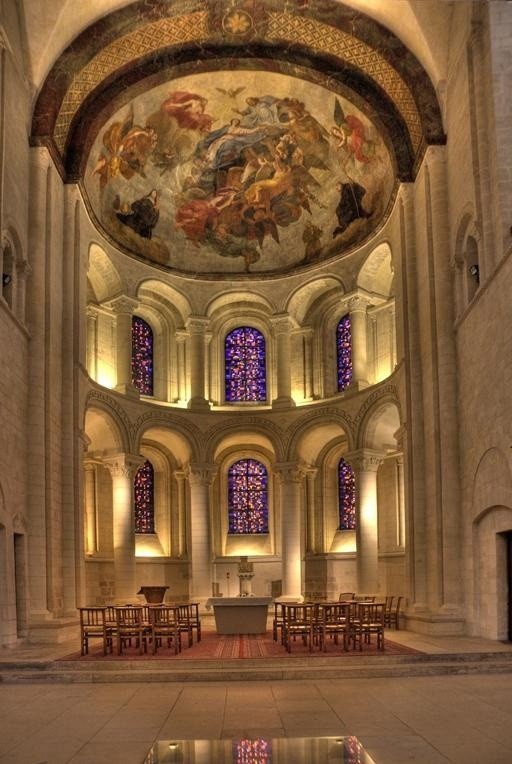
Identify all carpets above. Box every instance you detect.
[55,630,426,661]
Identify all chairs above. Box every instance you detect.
[77,602,201,655]
[273,592,404,655]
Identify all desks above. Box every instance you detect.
[206,596,273,635]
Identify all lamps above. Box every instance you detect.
[468,265,479,284]
[3,273,11,287]
